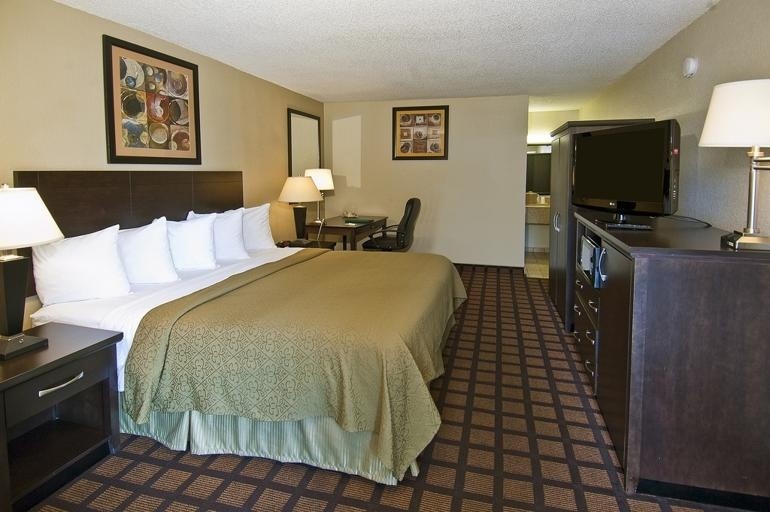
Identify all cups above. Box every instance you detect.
[342,207,358,218]
[146,94,189,125]
[149,122,169,144]
[120,91,146,119]
[144,64,167,93]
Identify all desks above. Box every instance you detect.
[304,216,388,251]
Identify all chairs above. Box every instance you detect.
[362,198,421,252]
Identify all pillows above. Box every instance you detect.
[151,211,217,272]
[186,209,251,261]
[118,215,179,286]
[31,224,131,308]
[224,203,277,252]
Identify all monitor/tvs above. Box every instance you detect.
[571,119,683,230]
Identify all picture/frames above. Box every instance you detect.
[392,105,449,160]
[102,34,202,165]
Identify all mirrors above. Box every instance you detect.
[526,143,552,195]
[287,107,321,205]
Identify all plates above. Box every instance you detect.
[121,59,144,89]
[167,71,188,96]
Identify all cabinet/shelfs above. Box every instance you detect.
[571,207,770,512]
[548,118,655,332]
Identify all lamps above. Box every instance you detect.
[697,78,770,251]
[278,176,324,247]
[305,169,334,223]
[0,187,65,361]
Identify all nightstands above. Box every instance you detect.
[275,239,336,251]
[0,322,124,512]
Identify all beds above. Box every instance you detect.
[12,170,468,486]
